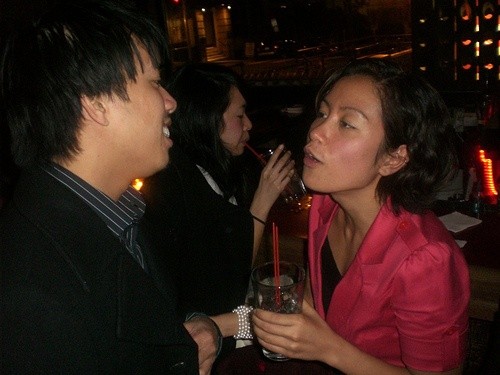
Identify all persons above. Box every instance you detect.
[157,62,295,316]
[208,58,472,375]
[0,0,224,375]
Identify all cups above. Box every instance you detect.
[252,261,306,360]
[256,148,310,213]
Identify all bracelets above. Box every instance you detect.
[191,316,223,356]
[232,305,256,340]
[252,215,267,225]
